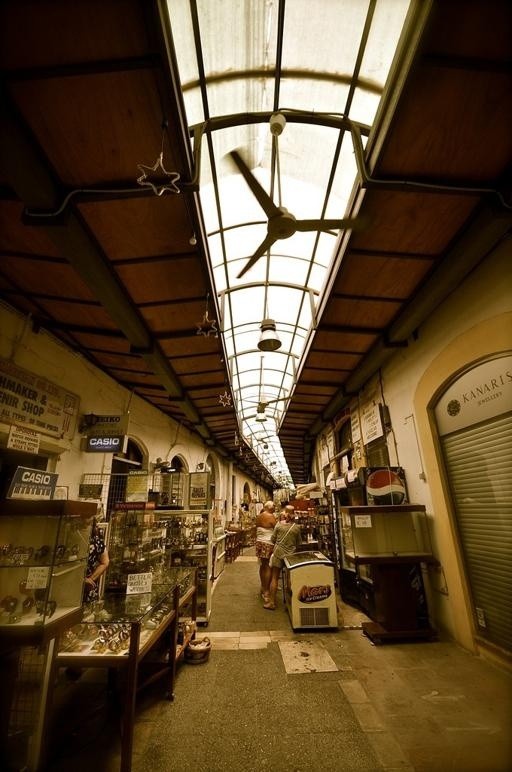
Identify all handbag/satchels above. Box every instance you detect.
[268,553,274,568]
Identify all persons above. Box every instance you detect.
[277,500,290,521]
[81,514,111,615]
[262,505,303,610]
[255,500,280,603]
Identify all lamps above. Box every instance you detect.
[257,299,282,352]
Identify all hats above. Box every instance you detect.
[280,505,297,518]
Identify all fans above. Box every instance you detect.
[229,114,361,279]
[239,364,293,409]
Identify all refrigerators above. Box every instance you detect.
[282,549,338,632]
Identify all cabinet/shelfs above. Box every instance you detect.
[0,497,214,771]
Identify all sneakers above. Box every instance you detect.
[261,591,277,610]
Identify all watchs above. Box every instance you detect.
[92,599,171,653]
[115,514,208,594]
[1,542,82,619]
